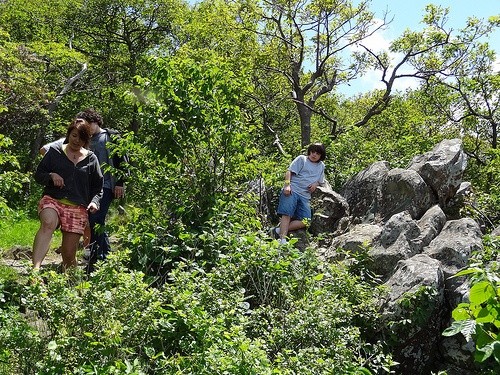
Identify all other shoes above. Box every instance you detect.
[270,227,280,241]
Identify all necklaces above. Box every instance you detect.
[71,150,76,159]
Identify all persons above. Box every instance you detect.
[31,119,104,273]
[271,143,326,244]
[77,107,129,278]
[39,137,90,245]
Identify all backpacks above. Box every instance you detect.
[104,128,130,186]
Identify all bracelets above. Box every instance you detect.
[284,180,290,184]
